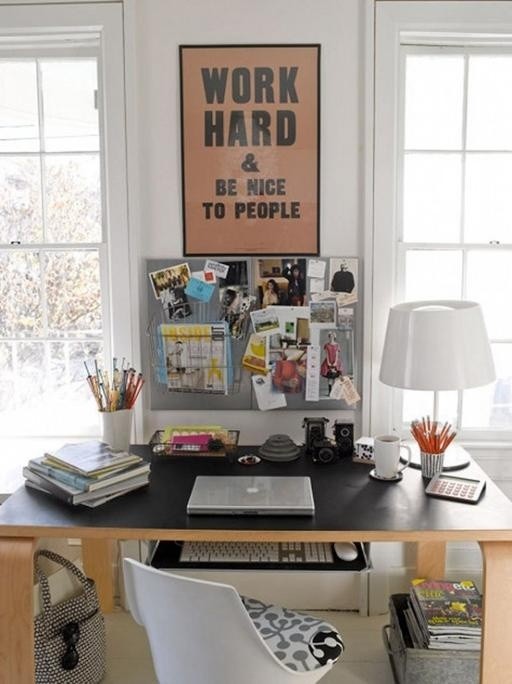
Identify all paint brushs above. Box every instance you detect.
[84,357,145,412]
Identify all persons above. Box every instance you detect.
[288,264,306,306]
[263,278,280,305]
[320,330,344,396]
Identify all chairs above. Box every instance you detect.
[121,554,346,682]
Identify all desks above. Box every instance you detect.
[1,444,511,683]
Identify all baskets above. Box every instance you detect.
[149,428,240,459]
[144,292,255,395]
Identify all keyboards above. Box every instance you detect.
[178,541,334,564]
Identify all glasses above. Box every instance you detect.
[61,622,80,670]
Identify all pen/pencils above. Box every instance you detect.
[409,415,457,454]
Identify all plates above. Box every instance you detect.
[369,468,404,481]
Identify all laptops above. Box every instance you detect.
[186,475,315,516]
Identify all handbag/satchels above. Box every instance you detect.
[33,549,107,684]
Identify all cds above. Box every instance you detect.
[237,455,261,465]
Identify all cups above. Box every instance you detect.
[97,408,133,453]
[374,435,412,478]
[420,451,445,478]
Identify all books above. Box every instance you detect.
[403,578,483,651]
[23,441,151,509]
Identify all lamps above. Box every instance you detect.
[380,299,511,470]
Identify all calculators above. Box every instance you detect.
[425,470,487,504]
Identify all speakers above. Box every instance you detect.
[305,417,325,455]
[335,419,354,458]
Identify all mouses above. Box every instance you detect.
[334,542,358,562]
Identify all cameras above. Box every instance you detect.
[312,438,336,464]
